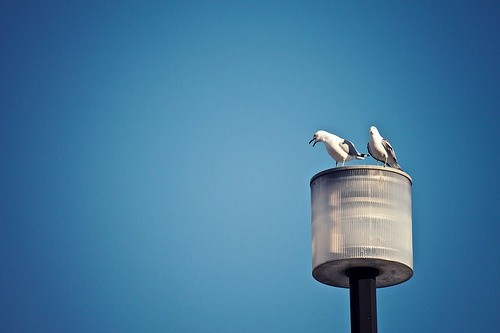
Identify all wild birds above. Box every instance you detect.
[368,126,404,172]
[308,130,370,168]
